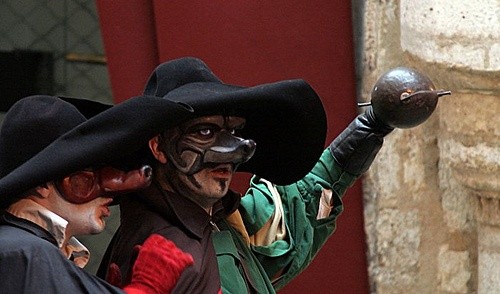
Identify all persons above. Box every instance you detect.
[96,58,453,294]
[0,94,195,294]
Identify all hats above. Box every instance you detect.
[143,56,328,185]
[0,80,194,210]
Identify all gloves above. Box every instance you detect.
[122,234,195,294]
[329,66,439,177]
